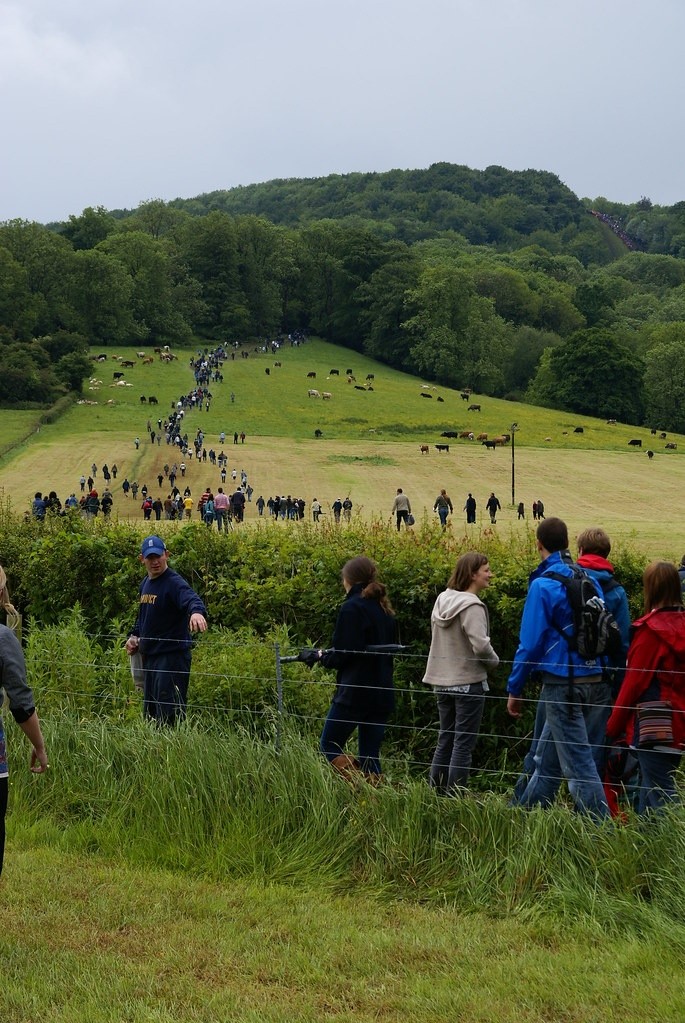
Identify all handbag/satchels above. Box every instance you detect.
[407,513,415,525]
[130,651,145,686]
[633,700,674,750]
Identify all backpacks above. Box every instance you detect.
[539,566,621,660]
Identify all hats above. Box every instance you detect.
[141,536,167,558]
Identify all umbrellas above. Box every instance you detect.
[278,642,414,666]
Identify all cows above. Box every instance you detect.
[322,392,331,399]
[307,372,316,378]
[87,346,178,386]
[421,388,472,402]
[627,429,677,459]
[140,395,145,403]
[149,396,158,404]
[308,389,320,398]
[315,429,322,437]
[435,445,450,452]
[606,419,617,424]
[468,404,481,412]
[330,367,375,391]
[419,445,429,452]
[441,430,510,449]
[545,426,583,442]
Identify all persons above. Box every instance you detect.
[420,553,499,797]
[189,328,305,386]
[392,488,411,532]
[267,495,305,521]
[486,492,501,524]
[126,536,208,730]
[433,489,453,532]
[256,496,265,516]
[310,497,321,523]
[506,518,685,827]
[343,498,352,522]
[332,499,342,523]
[517,502,525,519]
[24,462,254,535]
[320,558,399,786]
[133,387,246,468]
[0,565,47,877]
[463,493,477,524]
[533,500,545,519]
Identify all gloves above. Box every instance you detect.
[298,648,319,667]
